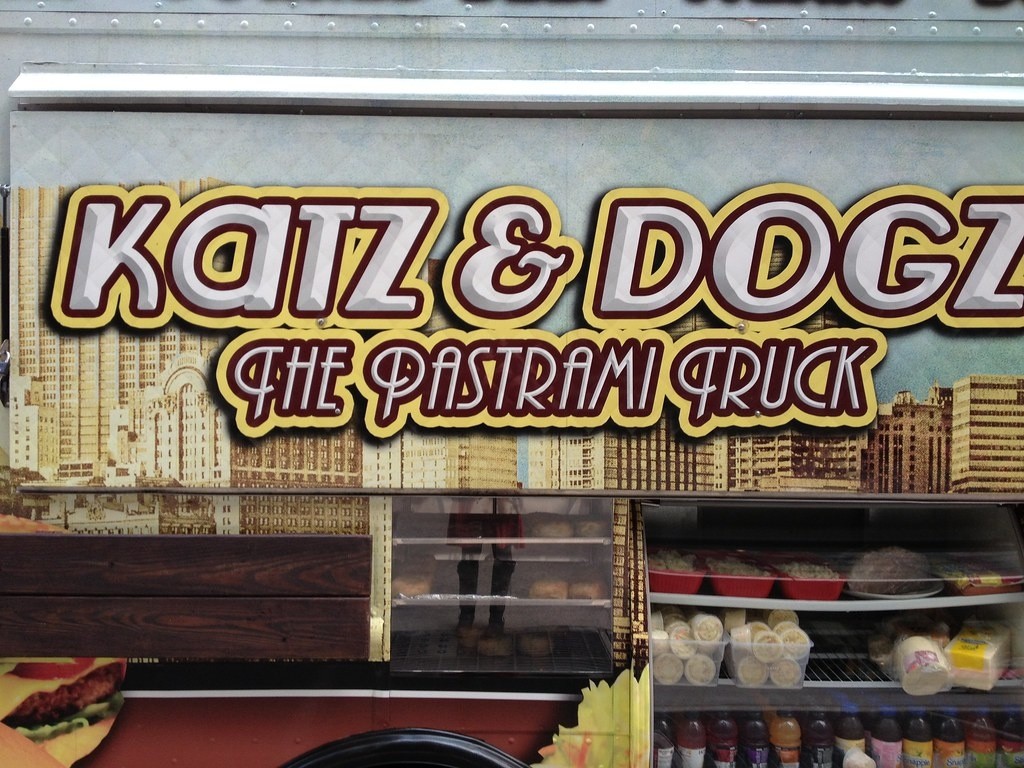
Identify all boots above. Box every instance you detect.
[486,561,516,639]
[457,562,479,638]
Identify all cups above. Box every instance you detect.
[843,747,877,768]
[896,636,949,696]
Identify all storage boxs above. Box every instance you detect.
[646,547,707,594]
[699,549,783,598]
[723,637,815,690]
[651,632,730,688]
[765,551,848,599]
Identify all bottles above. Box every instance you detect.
[742,712,834,768]
[966,710,1024,768]
[834,712,902,768]
[903,712,966,768]
[654,712,738,768]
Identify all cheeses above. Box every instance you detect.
[890,619,1014,696]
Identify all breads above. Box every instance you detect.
[391,515,609,658]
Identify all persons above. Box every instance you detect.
[446,497,526,639]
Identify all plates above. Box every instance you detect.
[843,583,945,599]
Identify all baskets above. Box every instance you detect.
[642,546,847,600]
